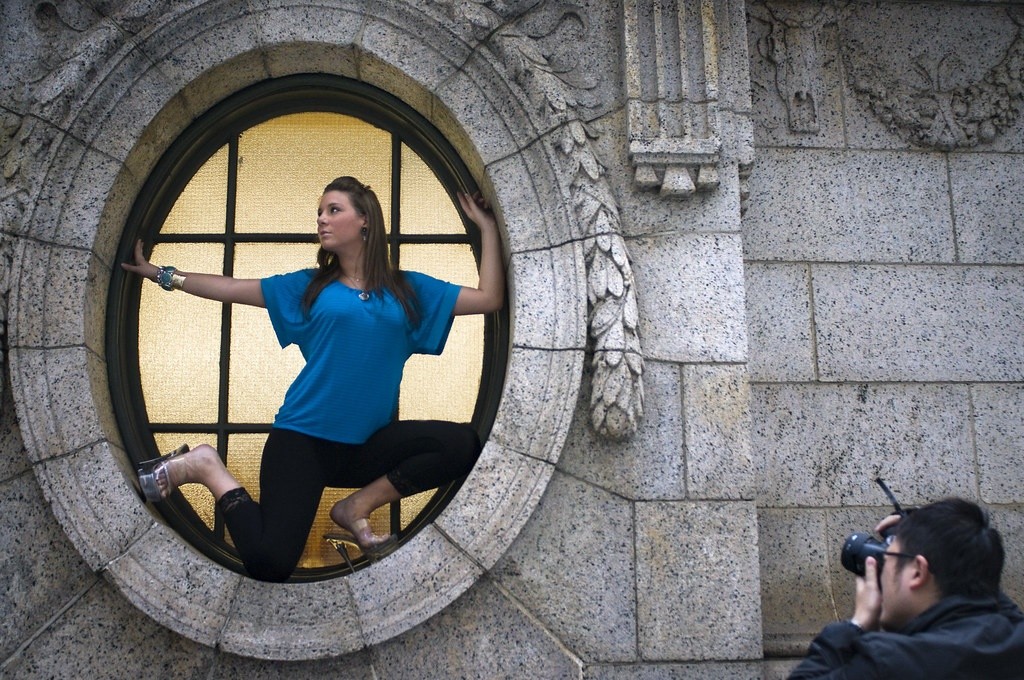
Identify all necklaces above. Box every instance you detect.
[345,274,372,300]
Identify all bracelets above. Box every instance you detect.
[158,266,188,291]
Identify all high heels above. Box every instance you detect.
[137,444,190,504]
[321,532,399,573]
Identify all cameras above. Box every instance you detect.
[841,524,897,590]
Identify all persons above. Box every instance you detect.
[120,177,504,580]
[788,496,1024,680]
